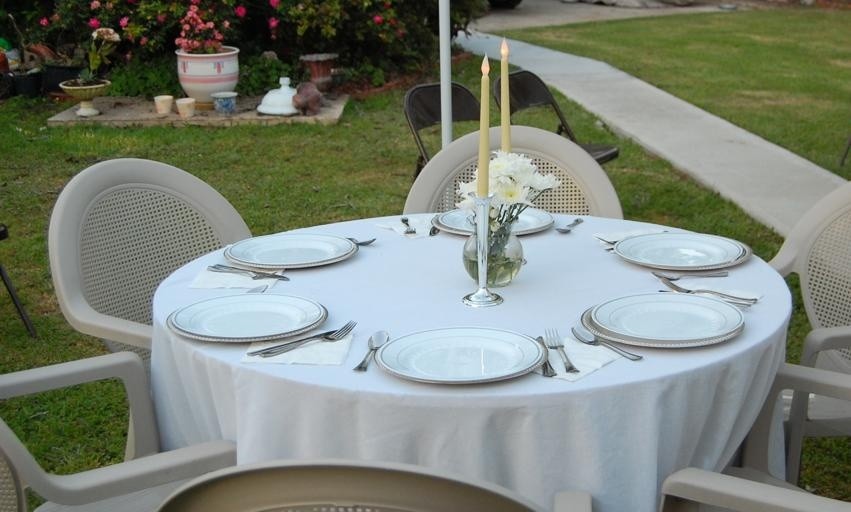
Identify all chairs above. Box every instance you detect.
[742,178,850,472]
[0,352,240,512]
[401,69,627,221]
[45,157,254,351]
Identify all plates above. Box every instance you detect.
[582,292,746,349]
[612,231,751,272]
[430,207,555,236]
[373,326,548,383]
[224,233,360,270]
[164,292,328,343]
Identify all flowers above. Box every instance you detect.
[176,3,224,54]
[78,27,120,84]
[454,151,564,282]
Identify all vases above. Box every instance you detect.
[300,52,339,93]
[155,44,241,120]
[58,78,111,118]
[461,223,524,288]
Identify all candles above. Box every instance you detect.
[478,52,492,196]
[499,38,513,151]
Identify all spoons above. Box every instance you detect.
[570,327,643,362]
[353,328,387,375]
[557,216,585,235]
[651,270,733,282]
[320,237,375,247]
[428,215,441,237]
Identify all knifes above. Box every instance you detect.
[246,328,348,358]
[532,333,557,379]
[209,266,291,285]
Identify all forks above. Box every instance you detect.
[660,279,755,306]
[596,236,616,248]
[207,263,277,281]
[545,328,580,376]
[401,215,418,236]
[248,321,360,358]
